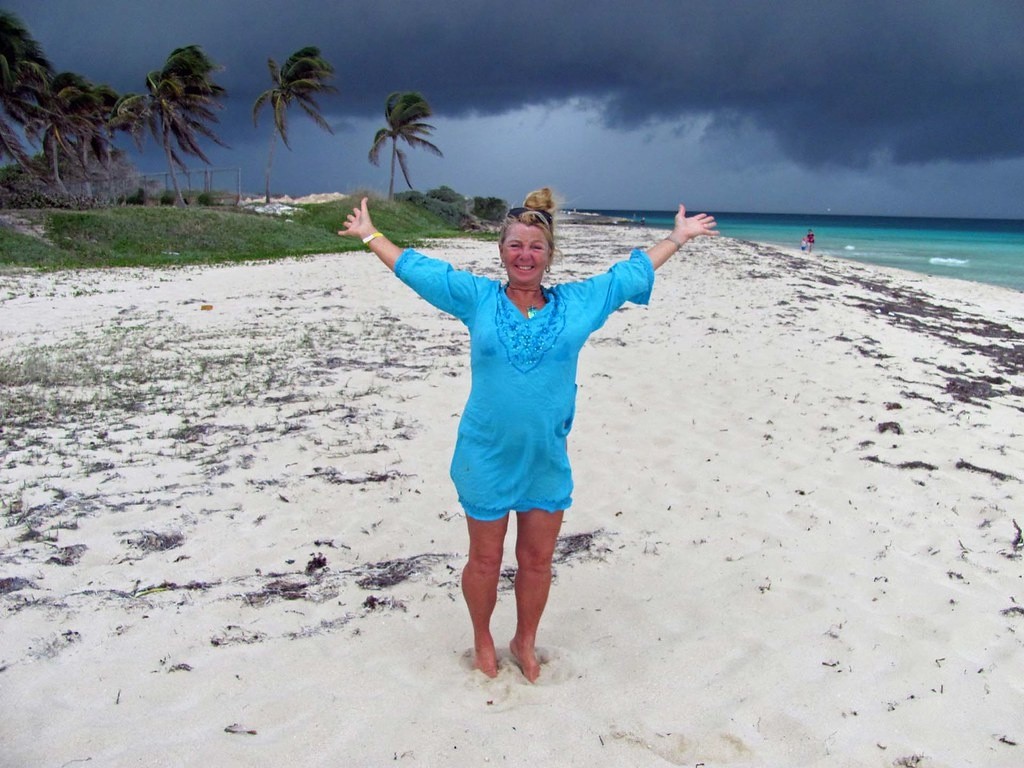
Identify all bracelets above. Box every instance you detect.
[664,235,682,250]
[363,231,384,248]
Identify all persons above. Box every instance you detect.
[801,228,815,252]
[337,188,720,686]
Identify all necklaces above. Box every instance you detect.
[511,296,546,318]
